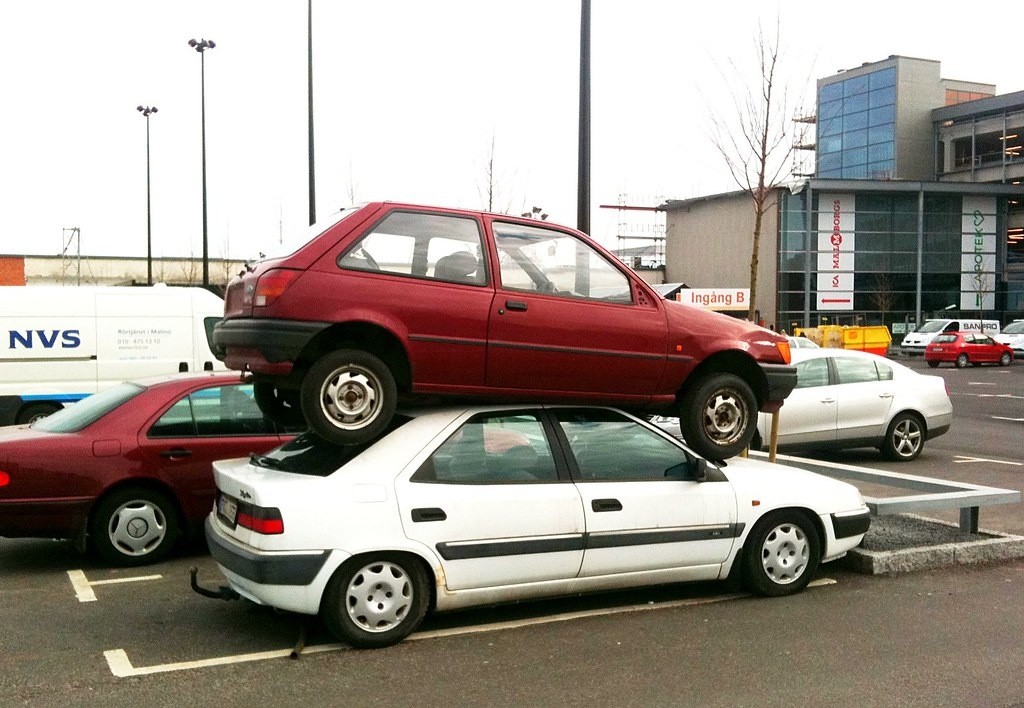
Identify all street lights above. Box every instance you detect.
[187,38,217,289]
[136,105,158,287]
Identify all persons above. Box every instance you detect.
[770,325,773,331]
[798,332,806,337]
[781,329,789,336]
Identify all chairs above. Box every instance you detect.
[433,251,478,283]
[449,437,542,482]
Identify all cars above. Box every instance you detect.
[743,335,954,462]
[924,331,1015,369]
[0,370,316,573]
[208,201,800,464]
[993,318,1024,356]
[204,402,872,651]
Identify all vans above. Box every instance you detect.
[899,318,1000,357]
[0,285,254,423]
